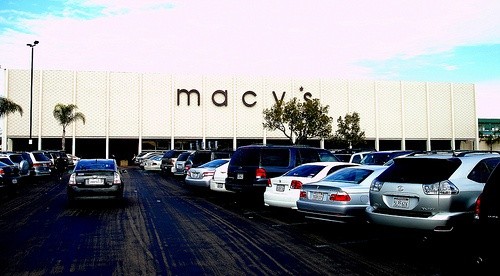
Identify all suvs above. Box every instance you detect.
[224,144,341,198]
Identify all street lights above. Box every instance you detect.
[27,40,39,150]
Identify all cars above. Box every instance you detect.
[263,161,362,212]
[133,149,235,194]
[325,147,416,166]
[296,164,390,227]
[473,159,500,237]
[364,149,500,233]
[0,149,81,187]
[66,158,127,210]
